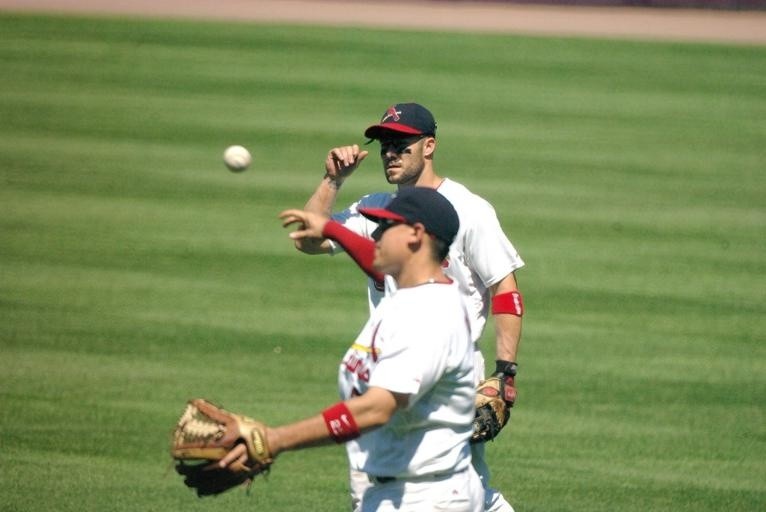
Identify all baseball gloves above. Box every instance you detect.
[473,374,516,444]
[173,398,273,497]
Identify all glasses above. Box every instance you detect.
[378,218,401,232]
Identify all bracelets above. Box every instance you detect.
[490,291,524,316]
[321,402,361,446]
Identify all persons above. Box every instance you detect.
[291,99,527,512]
[171,185,487,511]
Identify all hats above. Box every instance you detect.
[365,102,436,138]
[357,188,460,245]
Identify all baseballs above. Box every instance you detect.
[224,145,250,170]
